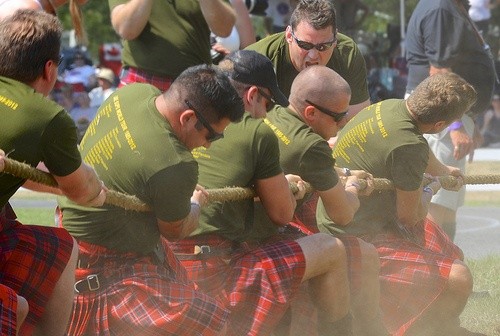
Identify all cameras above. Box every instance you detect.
[210,48,223,64]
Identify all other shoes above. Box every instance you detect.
[315,311,356,336]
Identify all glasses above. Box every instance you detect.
[52,53,65,67]
[183,98,225,143]
[257,87,278,113]
[289,31,336,51]
[305,99,348,123]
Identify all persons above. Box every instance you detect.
[467,0,490,38]
[108,0,237,95]
[404,0,494,247]
[0,0,88,19]
[0,11,107,336]
[60,63,245,336]
[207,0,274,67]
[51,56,118,135]
[477,51,500,136]
[316,73,478,336]
[244,0,370,147]
[181,49,353,336]
[265,64,381,336]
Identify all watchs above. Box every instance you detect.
[191,201,201,209]
[344,167,350,177]
[424,187,434,194]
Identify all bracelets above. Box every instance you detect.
[446,120,463,131]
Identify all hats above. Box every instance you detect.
[218,50,290,108]
[94,67,115,84]
